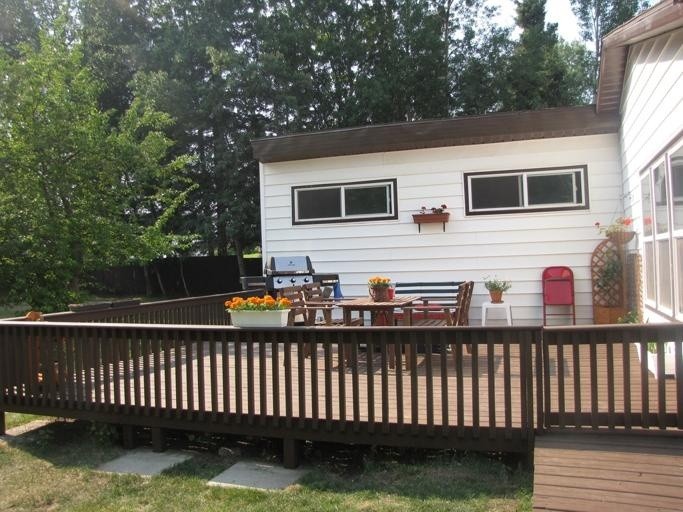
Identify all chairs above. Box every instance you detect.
[542,267,575,327]
[275,282,475,369]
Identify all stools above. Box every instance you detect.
[482,303,513,326]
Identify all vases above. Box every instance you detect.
[607,232,635,244]
[225,309,291,328]
[412,214,449,223]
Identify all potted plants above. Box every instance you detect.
[39,336,73,387]
[617,312,676,381]
[482,273,511,304]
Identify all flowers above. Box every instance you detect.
[368,277,391,286]
[644,217,667,235]
[223,295,292,310]
[596,216,633,236]
[419,205,447,214]
